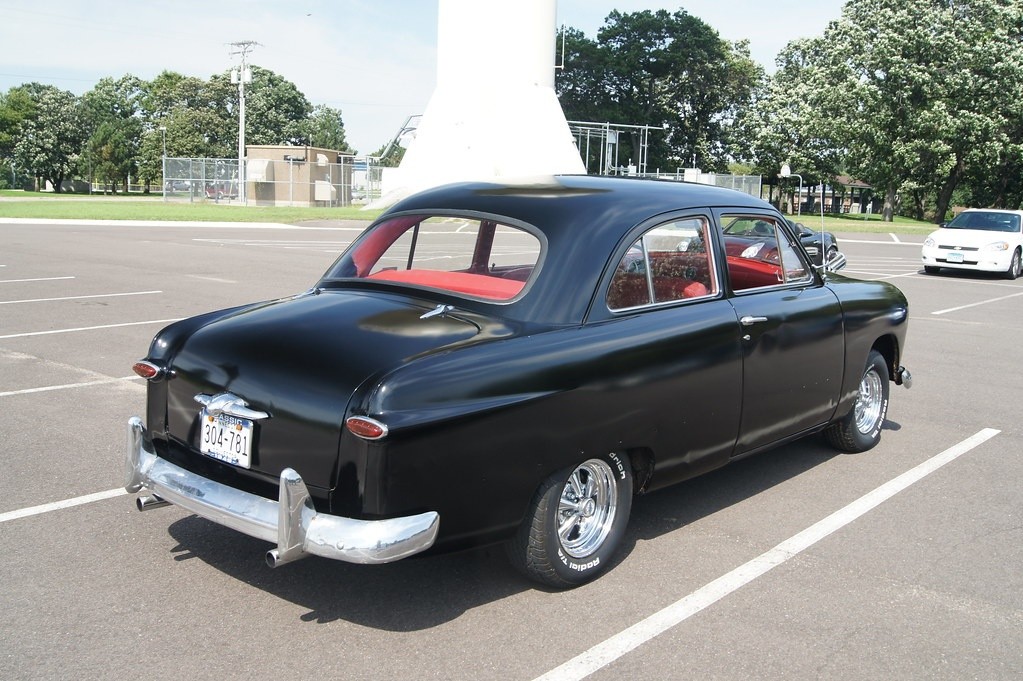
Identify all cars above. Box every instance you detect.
[676,216,847,277]
[920,205,1023,279]
[125,170,909,586]
[350,190,363,200]
[164,179,190,191]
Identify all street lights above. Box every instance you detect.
[204,137,209,158]
[159,127,166,157]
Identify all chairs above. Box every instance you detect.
[630,274,707,305]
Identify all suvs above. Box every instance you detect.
[203,181,238,200]
